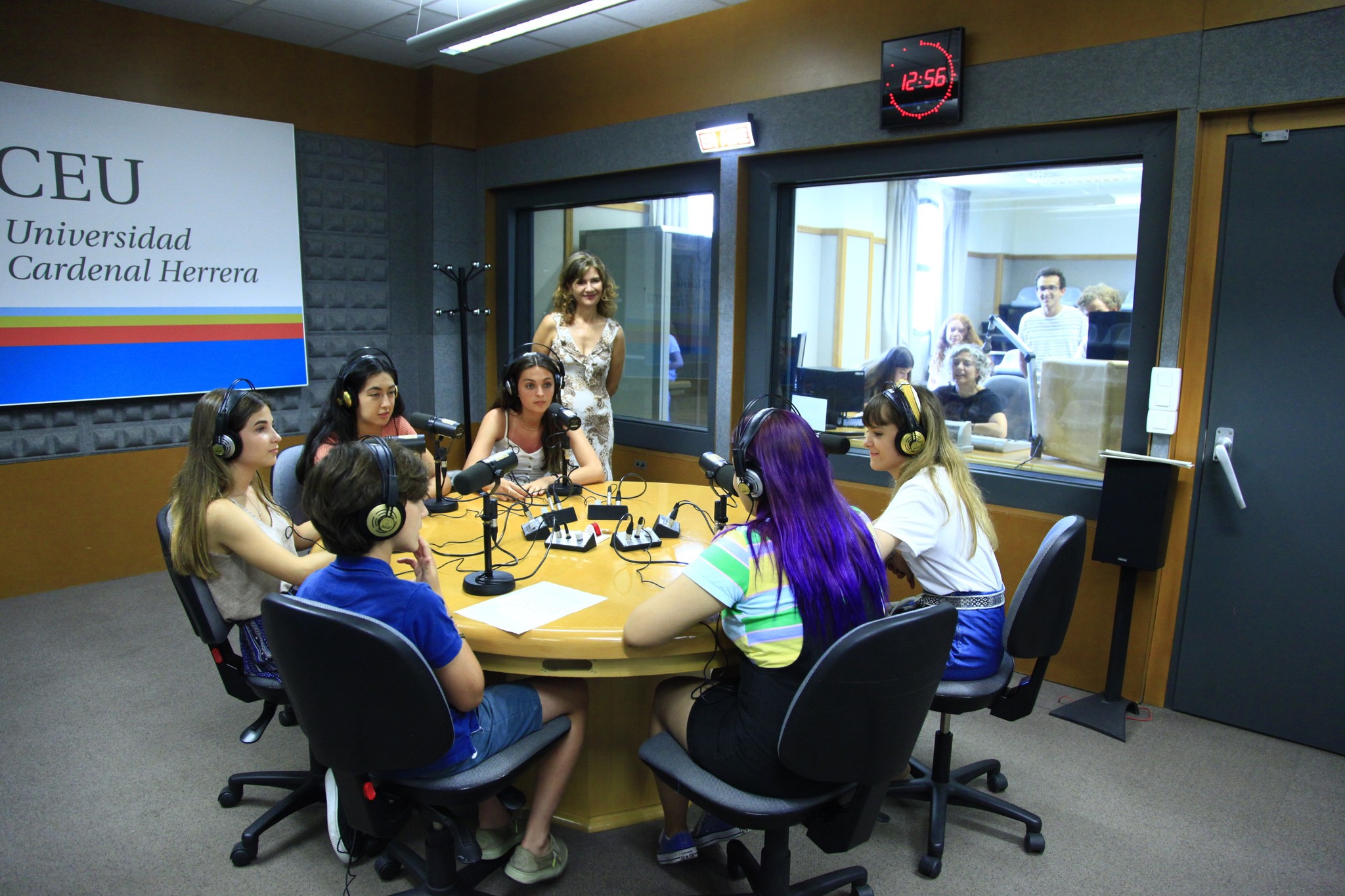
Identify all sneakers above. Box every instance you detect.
[476,814,528,860]
[692,808,752,849]
[657,827,698,865]
[505,831,568,884]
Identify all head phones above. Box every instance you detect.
[501,342,566,397]
[210,378,256,462]
[732,394,802,498]
[335,346,398,408]
[356,434,407,541]
[882,381,928,457]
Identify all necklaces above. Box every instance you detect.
[518,414,543,430]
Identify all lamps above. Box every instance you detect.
[694,110,757,153]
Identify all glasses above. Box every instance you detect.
[1037,287,1062,292]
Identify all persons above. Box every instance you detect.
[928,343,1008,439]
[168,386,339,689]
[1017,267,1090,398]
[461,352,605,501]
[858,346,914,412]
[293,347,452,501]
[619,406,889,865]
[1076,284,1123,318]
[286,436,587,885]
[842,383,1007,681]
[667,334,684,422]
[927,314,992,393]
[531,251,626,481]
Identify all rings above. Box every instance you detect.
[509,489,512,494]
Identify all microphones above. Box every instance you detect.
[815,432,851,455]
[548,402,582,430]
[358,433,426,454]
[453,449,519,495]
[982,321,995,354]
[698,451,739,496]
[408,411,465,439]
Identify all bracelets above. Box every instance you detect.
[458,633,465,638]
[551,473,560,484]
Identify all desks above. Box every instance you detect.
[879,513,1091,878]
[308,481,756,833]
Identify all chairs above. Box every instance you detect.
[155,500,298,743]
[638,601,961,896]
[217,593,497,896]
[986,376,1032,448]
